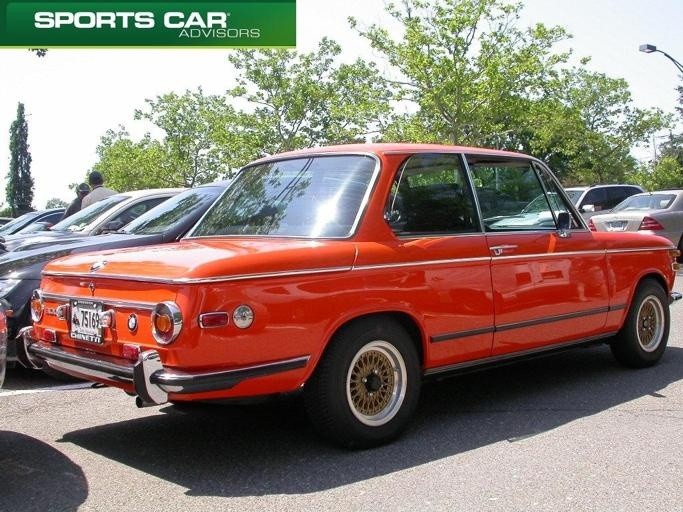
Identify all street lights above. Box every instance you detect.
[638,44,683,76]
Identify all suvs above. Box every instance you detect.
[488,181,648,232]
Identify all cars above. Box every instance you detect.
[15,138,682,451]
[589,187,683,267]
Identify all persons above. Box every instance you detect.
[81,171,120,210]
[61,183,90,221]
